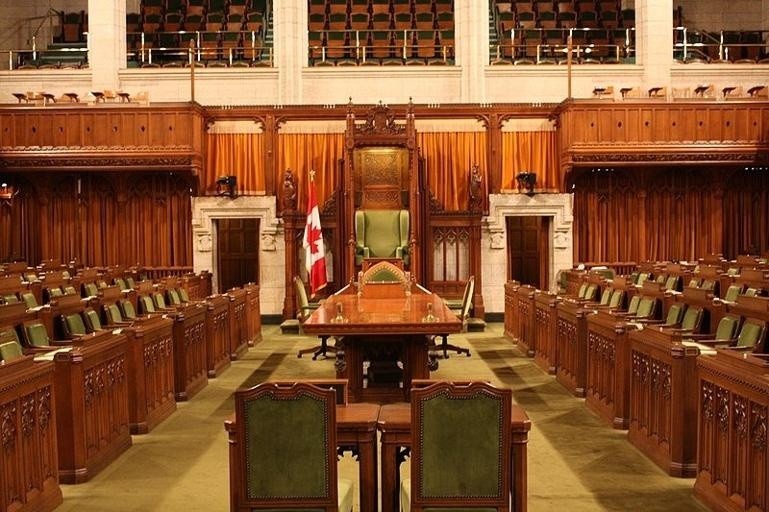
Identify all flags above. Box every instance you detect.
[302,177,328,299]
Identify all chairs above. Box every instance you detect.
[292,275,341,363]
[268,379,348,405]
[430,275,477,360]
[357,265,412,300]
[401,382,512,512]
[234,380,354,512]
[411,380,489,413]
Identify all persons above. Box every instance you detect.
[468,165,483,210]
[282,167,296,199]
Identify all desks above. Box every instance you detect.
[378,403,532,510]
[224,403,381,512]
[303,293,464,403]
